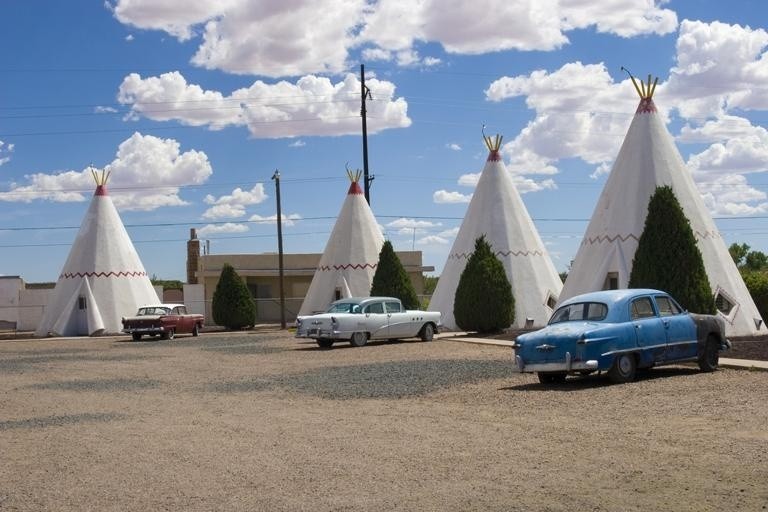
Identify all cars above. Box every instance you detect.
[120,302,206,342]
[292,294,442,350]
[507,288,732,387]
